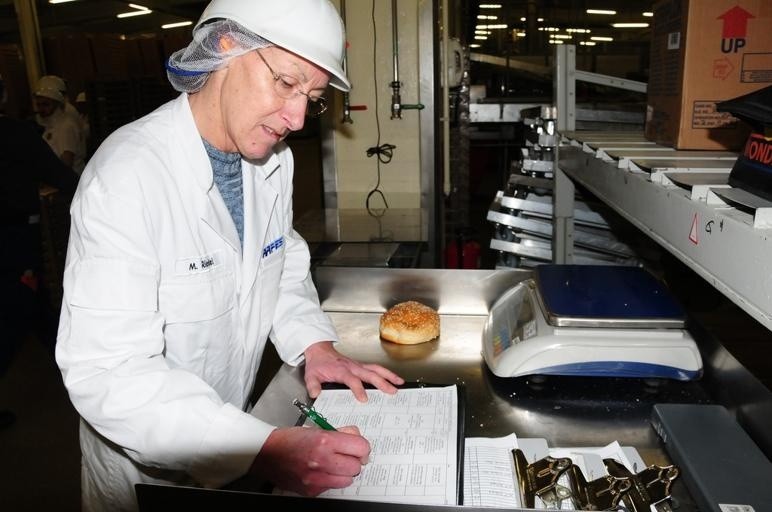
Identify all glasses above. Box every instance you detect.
[246,39,329,118]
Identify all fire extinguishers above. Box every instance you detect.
[459,230,483,269]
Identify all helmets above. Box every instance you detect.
[32,74,67,106]
[191,0,352,94]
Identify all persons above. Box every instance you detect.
[53,0,404,511]
[31,73,87,169]
[1,81,80,433]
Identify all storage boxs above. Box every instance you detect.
[643,0,772,151]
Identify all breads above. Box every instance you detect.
[379,300,441,346]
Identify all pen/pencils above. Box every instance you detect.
[292,398,338,430]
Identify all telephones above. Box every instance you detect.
[439,38,465,89]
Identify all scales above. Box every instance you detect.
[482,264,706,392]
